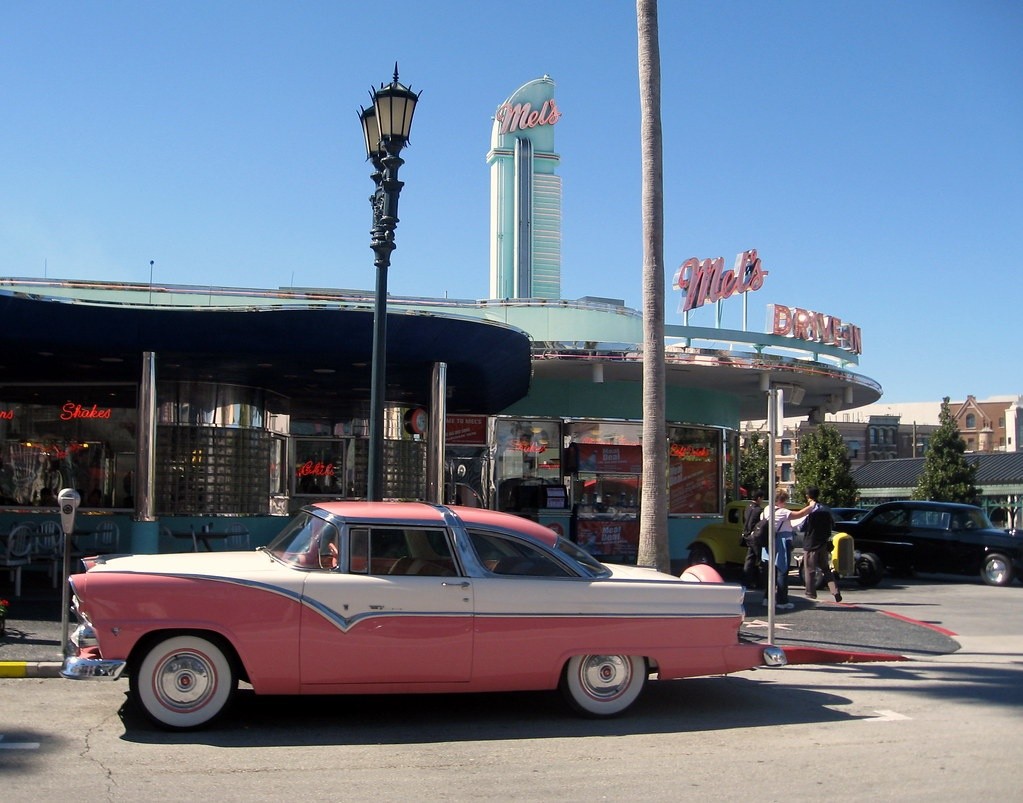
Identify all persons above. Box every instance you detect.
[759,489,816,610]
[795,485,842,603]
[743,490,768,591]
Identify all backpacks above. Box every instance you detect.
[753,508,784,547]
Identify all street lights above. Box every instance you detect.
[353,58,423,503]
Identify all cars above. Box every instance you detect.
[685,498,853,591]
[827,504,878,538]
[57,502,787,743]
[838,500,1023,587]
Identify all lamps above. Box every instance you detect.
[790,386,805,405]
[773,383,793,403]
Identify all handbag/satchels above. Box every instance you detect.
[739,533,746,547]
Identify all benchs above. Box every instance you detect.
[0,547,112,566]
[483,557,546,574]
[388,556,455,576]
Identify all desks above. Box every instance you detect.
[176,531,247,550]
[0,529,112,596]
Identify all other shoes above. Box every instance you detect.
[805,593,816,599]
[776,603,794,609]
[762,599,768,606]
[834,593,842,602]
